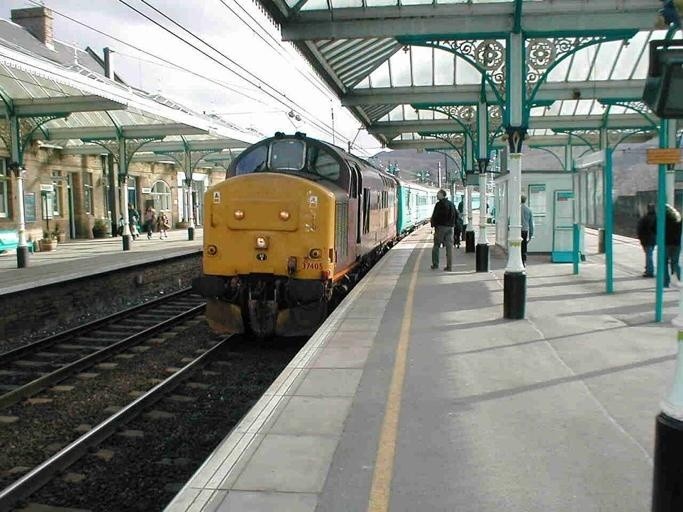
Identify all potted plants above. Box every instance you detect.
[38,223,64,252]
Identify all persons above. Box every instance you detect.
[664,203,683,289]
[430,188,459,272]
[120,202,170,241]
[437,194,490,241]
[637,202,657,277]
[508,195,534,271]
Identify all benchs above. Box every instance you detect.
[0,228,32,255]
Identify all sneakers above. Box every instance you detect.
[443,267,451,272]
[430,262,438,268]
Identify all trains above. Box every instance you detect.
[199,132,494,339]
